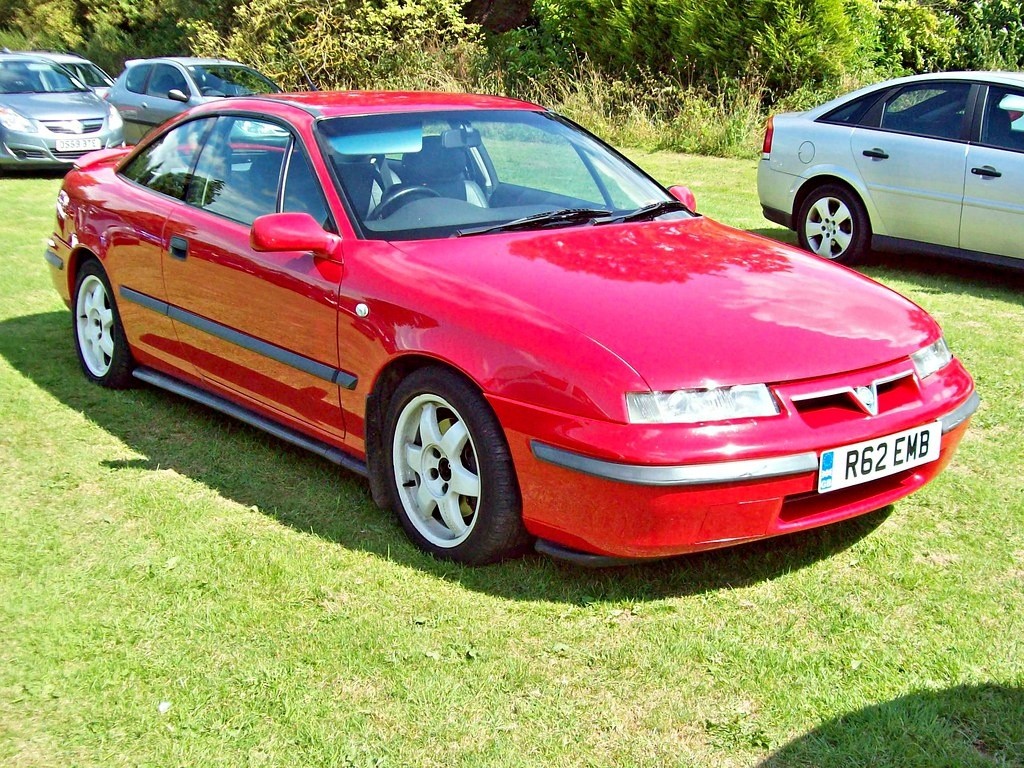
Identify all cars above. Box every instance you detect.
[101,55,290,148]
[0,50,126,171]
[755,71,1024,271]
[2,47,117,98]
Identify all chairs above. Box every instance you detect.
[155,74,177,95]
[291,144,386,224]
[205,72,226,94]
[403,135,491,210]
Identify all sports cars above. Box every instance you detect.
[44,88,982,569]
